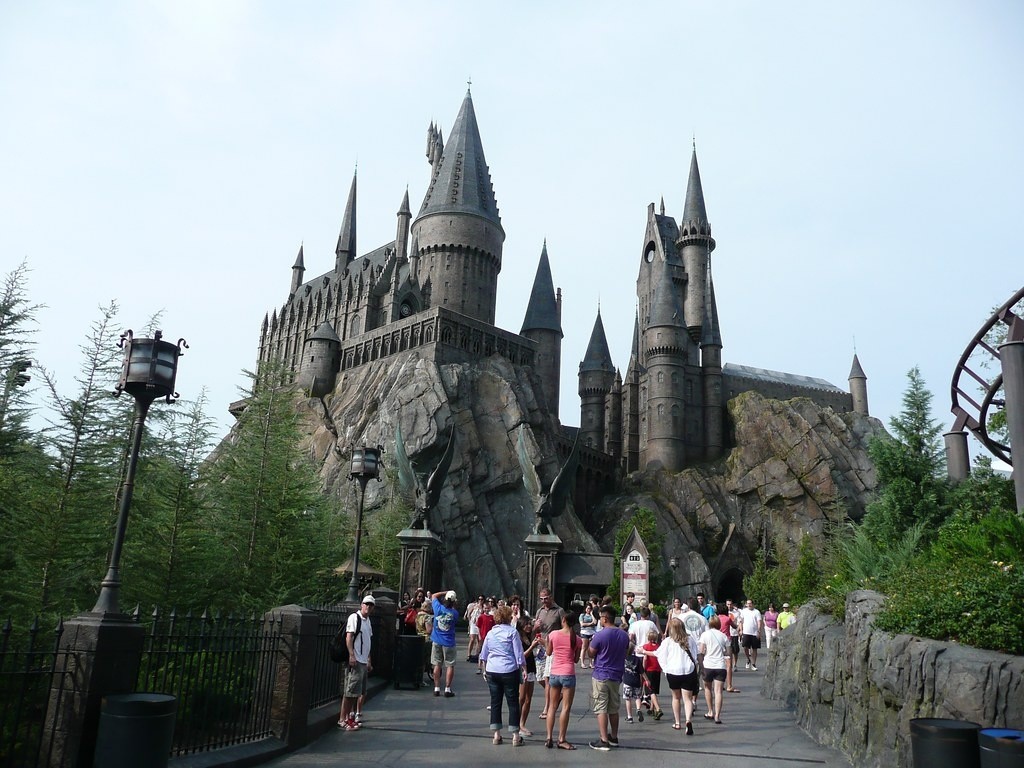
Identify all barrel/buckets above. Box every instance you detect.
[909,718,982,768]
[392,635,425,684]
[93,692,178,768]
[977,727,1024,768]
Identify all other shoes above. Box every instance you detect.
[624,717,633,724]
[751,664,759,671]
[444,689,455,698]
[654,710,664,721]
[646,710,655,716]
[745,660,751,669]
[433,690,440,697]
[637,709,644,722]
[642,699,651,709]
[581,664,595,669]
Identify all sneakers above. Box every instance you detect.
[600,734,619,747]
[337,718,350,731]
[347,718,359,730]
[355,713,364,727]
[589,739,611,751]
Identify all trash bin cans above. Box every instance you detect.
[978,728,1024,768]
[394,635,426,690]
[909,718,982,768]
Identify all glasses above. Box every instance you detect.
[363,602,374,606]
[540,594,552,601]
[479,599,483,600]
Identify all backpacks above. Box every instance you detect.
[329,612,362,662]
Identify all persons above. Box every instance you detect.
[336,594,375,730]
[401,586,795,752]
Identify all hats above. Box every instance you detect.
[782,603,790,607]
[445,590,456,601]
[640,598,649,607]
[363,595,375,605]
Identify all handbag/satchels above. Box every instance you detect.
[405,609,417,624]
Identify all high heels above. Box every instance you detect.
[493,736,503,745]
[545,739,553,749]
[556,740,577,750]
[513,737,525,747]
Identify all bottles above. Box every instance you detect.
[532,633,542,656]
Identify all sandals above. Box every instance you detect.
[672,723,681,730]
[685,720,695,736]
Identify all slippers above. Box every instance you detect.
[539,713,547,719]
[714,719,722,724]
[727,688,740,693]
[703,713,714,720]
